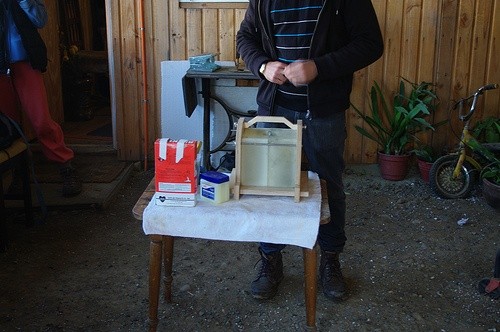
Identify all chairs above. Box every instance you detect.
[181,66,264,170]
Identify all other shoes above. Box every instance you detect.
[63,160,81,196]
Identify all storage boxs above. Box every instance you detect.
[154,137,198,207]
[199,171,230,203]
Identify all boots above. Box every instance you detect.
[319,251,347,300]
[250,248,283,298]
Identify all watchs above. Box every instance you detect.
[259,64,266,73]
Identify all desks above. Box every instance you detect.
[0,149,33,253]
[131,170,331,332]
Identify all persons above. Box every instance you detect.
[0,0,83,198]
[235,0,384,301]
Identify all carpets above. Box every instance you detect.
[31,150,129,183]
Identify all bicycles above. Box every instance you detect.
[428,83,500,199]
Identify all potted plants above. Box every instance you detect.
[461,116,500,209]
[350,79,451,183]
